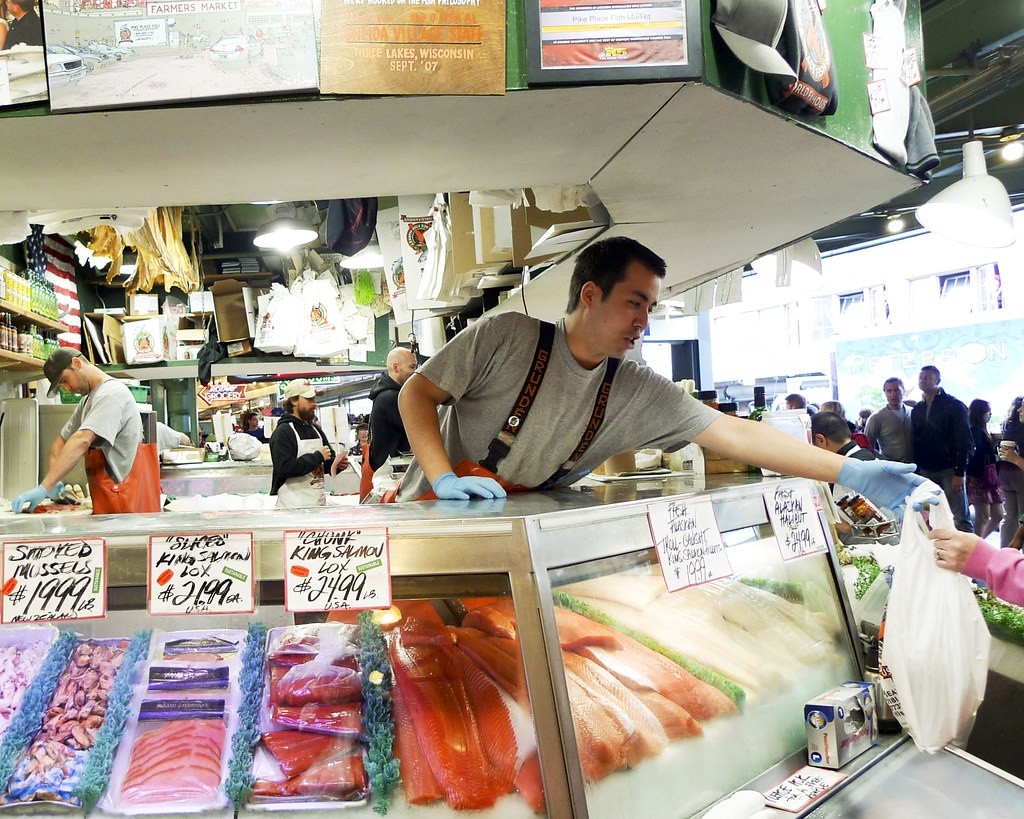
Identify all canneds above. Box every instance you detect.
[690,390,739,418]
[837,495,891,534]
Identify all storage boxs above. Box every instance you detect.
[126,384,151,404]
[129,293,160,315]
[178,344,203,360]
[209,279,257,345]
[162,447,206,464]
[188,290,215,312]
[83,312,126,365]
[57,387,84,404]
[120,319,166,363]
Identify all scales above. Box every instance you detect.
[162,447,206,463]
[390,449,414,480]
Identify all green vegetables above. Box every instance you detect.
[354,269,376,306]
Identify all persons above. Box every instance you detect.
[348,424,369,457]
[11,347,161,515]
[269,378,349,509]
[784,394,875,497]
[863,366,1024,552]
[928,528,1024,607]
[394,236,941,511]
[243,413,266,444]
[368,347,418,472]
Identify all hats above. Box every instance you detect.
[284,378,324,402]
[43,347,82,399]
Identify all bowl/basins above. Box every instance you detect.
[204,453,219,462]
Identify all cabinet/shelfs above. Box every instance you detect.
[0,299,70,373]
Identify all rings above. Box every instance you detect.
[936,550,941,559]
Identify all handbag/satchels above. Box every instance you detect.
[882,478,991,755]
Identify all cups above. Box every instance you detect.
[1000,441,1015,452]
[864,652,902,734]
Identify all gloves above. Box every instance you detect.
[11,484,48,513]
[837,454,942,520]
[432,473,507,500]
[48,480,65,501]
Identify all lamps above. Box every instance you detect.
[253,201,319,248]
[916,109,1018,254]
[750,236,823,285]
[328,229,385,270]
[997,127,1024,161]
[882,211,906,234]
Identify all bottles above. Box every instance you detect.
[0,269,59,361]
[836,495,872,534]
[718,403,738,417]
[748,387,768,473]
[848,495,891,534]
[698,390,718,410]
